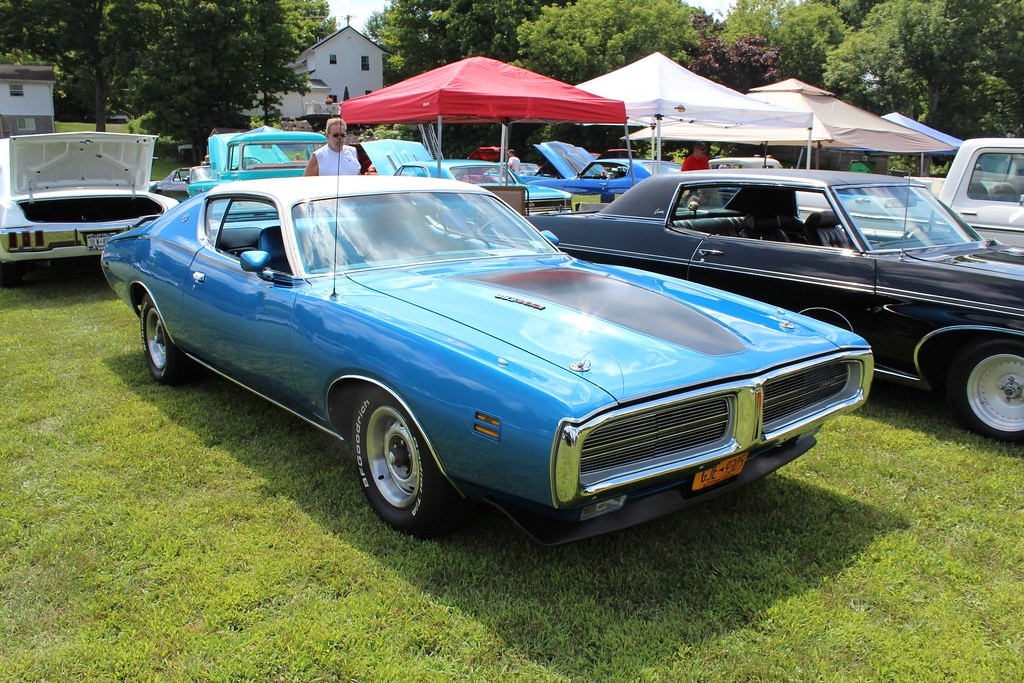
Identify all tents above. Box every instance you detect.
[336,53,638,207]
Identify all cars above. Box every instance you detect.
[587,149,639,160]
[708,157,783,169]
[187,131,328,197]
[455,146,541,184]
[520,167,1024,443]
[150,168,191,203]
[502,140,683,211]
[360,139,573,214]
[103,176,875,548]
[0,130,179,288]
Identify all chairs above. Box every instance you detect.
[211,226,262,258]
[258,225,292,276]
[968,164,991,199]
[803,212,849,250]
[738,212,791,242]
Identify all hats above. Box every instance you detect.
[508,149,515,153]
[693,142,705,148]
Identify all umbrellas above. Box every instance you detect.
[572,45,974,184]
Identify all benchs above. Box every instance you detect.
[245,161,308,170]
[671,216,743,237]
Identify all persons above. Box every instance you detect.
[967,164,990,200]
[507,149,521,177]
[303,117,362,176]
[681,141,710,206]
[292,153,302,162]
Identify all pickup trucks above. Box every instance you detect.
[795,138,1024,250]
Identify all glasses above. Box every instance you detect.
[697,147,707,150]
[334,134,345,137]
